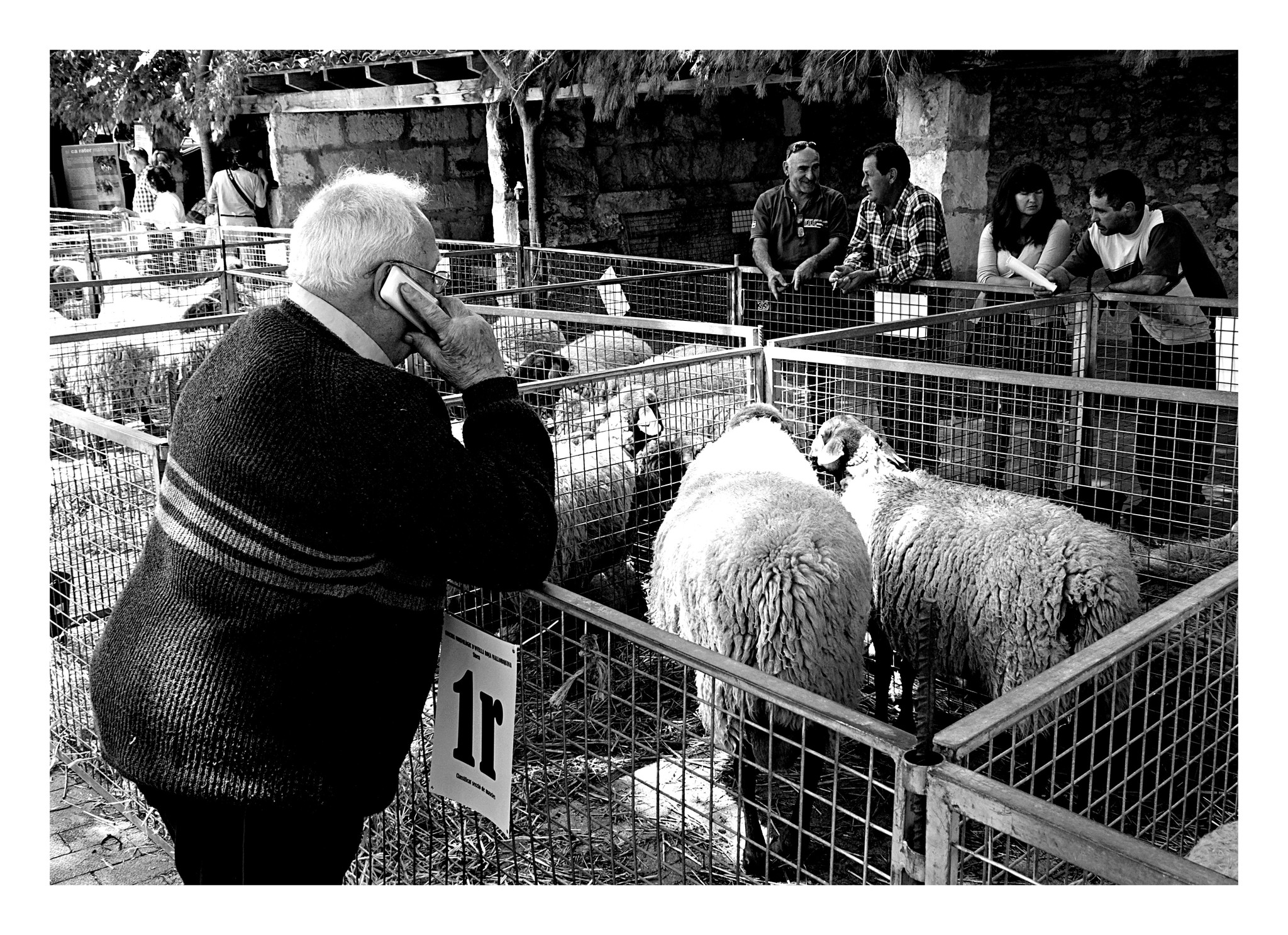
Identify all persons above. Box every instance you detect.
[975,160,1070,501]
[207,150,271,290]
[151,150,174,171]
[126,147,157,215]
[829,141,952,477]
[88,161,560,885]
[110,166,196,289]
[749,141,849,443]
[1031,168,1232,544]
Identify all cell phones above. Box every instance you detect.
[379,265,439,334]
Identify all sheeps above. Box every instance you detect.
[813,413,1145,730]
[648,403,870,871]
[49,259,279,440]
[488,316,798,586]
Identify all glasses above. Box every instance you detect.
[164,162,170,169]
[364,260,451,294]
[787,141,818,161]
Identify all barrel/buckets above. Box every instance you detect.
[50,571,71,651]
